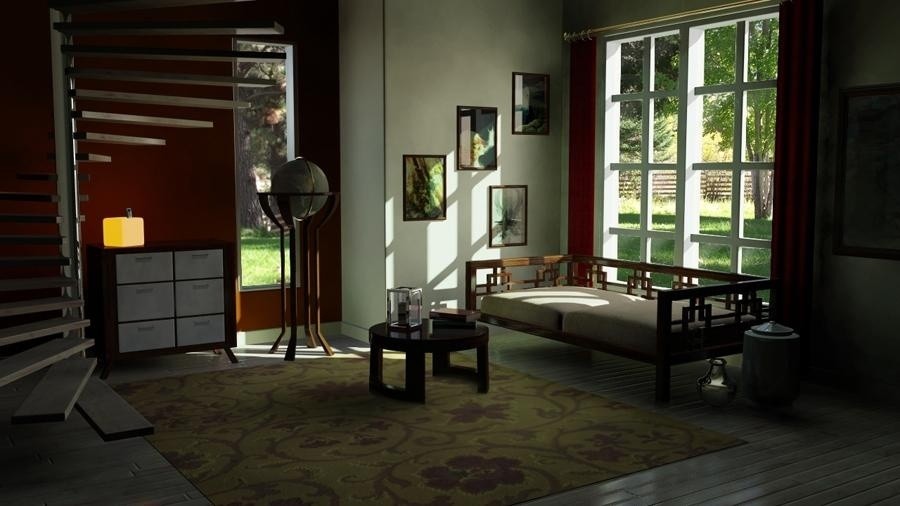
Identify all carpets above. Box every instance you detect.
[106,348,747,506]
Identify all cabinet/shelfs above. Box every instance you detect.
[84,240,239,381]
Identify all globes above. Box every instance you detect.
[257,157,339,361]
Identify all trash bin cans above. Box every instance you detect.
[743,320,801,411]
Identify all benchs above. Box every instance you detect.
[464,252,771,401]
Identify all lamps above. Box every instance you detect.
[102,207,146,249]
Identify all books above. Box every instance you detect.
[427,307,483,323]
[432,318,477,330]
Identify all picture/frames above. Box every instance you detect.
[832,81,898,262]
[511,71,550,135]
[402,153,447,223]
[489,185,528,247]
[456,105,498,171]
